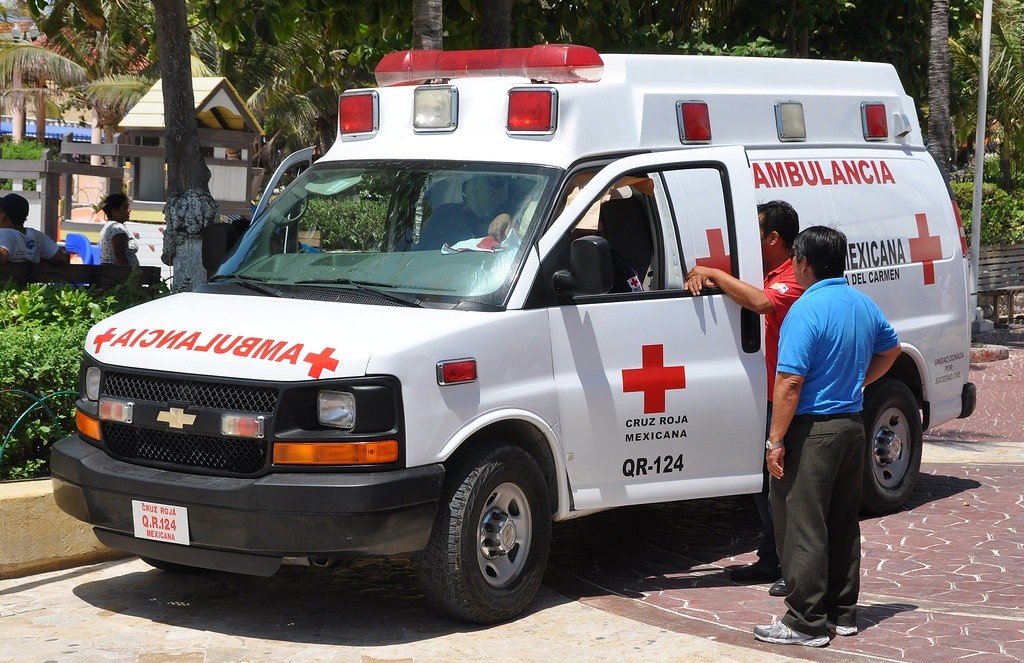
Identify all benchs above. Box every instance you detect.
[967,243,1024,331]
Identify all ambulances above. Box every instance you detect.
[49,45,978,624]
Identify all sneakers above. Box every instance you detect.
[752,615,829,647]
[825,620,858,636]
[728,561,777,584]
[769,578,788,596]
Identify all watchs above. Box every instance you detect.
[766,439,784,450]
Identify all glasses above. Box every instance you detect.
[789,251,797,260]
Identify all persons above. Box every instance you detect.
[0,193,69,265]
[683,201,807,594]
[751,225,901,646]
[99,193,139,266]
[487,179,604,242]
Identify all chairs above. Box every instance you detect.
[413,202,480,251]
[597,198,651,293]
